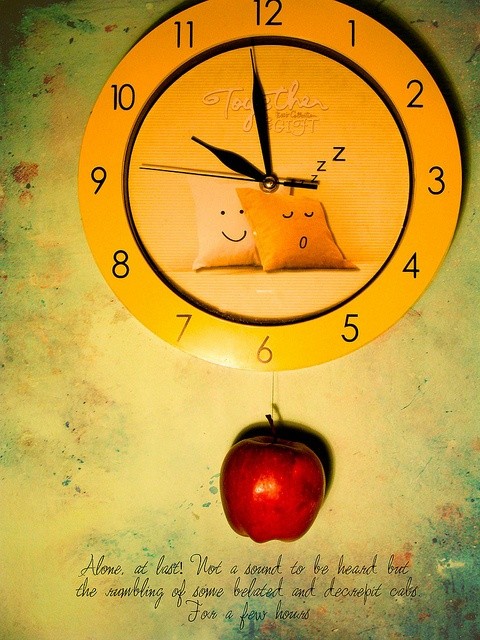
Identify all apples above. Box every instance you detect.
[219,415,325,543]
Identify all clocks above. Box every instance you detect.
[77,0,464,374]
